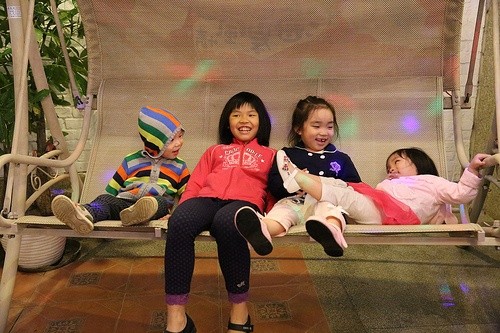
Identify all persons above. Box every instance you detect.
[234,96,361,256]
[52,105,190,235]
[165,92,277,333]
[276,148,492,224]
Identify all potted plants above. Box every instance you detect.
[0,0,88,269]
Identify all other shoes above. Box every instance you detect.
[164,312,197,333]
[119,196,159,226]
[306,215,348,257]
[276,149,301,194]
[227,314,254,333]
[234,205,274,256]
[51,195,94,234]
